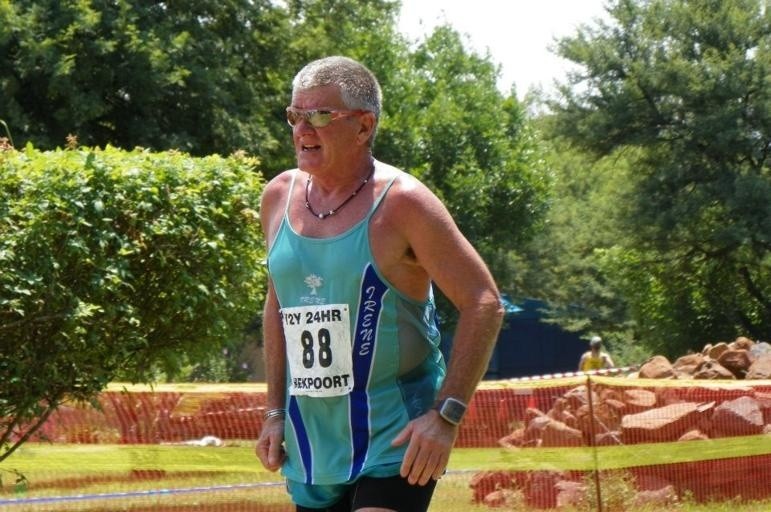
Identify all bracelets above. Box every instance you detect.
[263,407,287,421]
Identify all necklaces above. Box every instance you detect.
[304,165,376,220]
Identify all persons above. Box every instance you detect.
[579,335,615,371]
[255,53,508,512]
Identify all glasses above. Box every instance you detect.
[281,103,367,131]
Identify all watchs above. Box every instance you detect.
[430,396,468,426]
